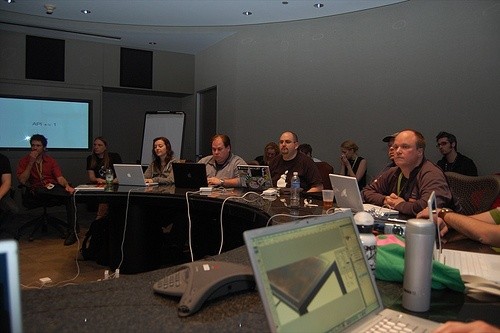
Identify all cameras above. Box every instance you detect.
[382,220,407,237]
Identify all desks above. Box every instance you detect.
[267,256,347,316]
[20,183,499,333]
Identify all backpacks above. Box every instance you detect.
[81,213,129,267]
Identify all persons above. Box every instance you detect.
[17,134,80,246]
[1,153,18,225]
[268,131,324,194]
[196,134,251,188]
[297,144,323,162]
[435,131,478,177]
[338,141,367,191]
[361,129,464,217]
[375,132,397,179]
[252,142,280,167]
[86,137,123,186]
[417,205,500,246]
[143,137,176,185]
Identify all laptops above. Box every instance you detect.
[242,208,448,332]
[235,163,275,190]
[73,182,108,193]
[0,238,24,332]
[112,162,159,187]
[328,172,401,218]
[427,189,500,283]
[170,161,210,192]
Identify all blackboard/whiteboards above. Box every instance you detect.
[140,110,186,167]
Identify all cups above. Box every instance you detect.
[321,189,334,208]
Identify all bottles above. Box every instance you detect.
[291,172,300,206]
[354,211,378,282]
[403,219,435,312]
[106,169,113,189]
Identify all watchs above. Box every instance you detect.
[220,178,224,185]
[438,208,453,219]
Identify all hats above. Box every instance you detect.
[383,133,401,142]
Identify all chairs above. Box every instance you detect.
[15,185,77,243]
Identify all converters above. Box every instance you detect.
[334,206,353,212]
[199,186,212,191]
[262,186,278,197]
[103,268,110,279]
[39,275,53,284]
[115,268,119,278]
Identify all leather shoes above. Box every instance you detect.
[63,231,80,246]
